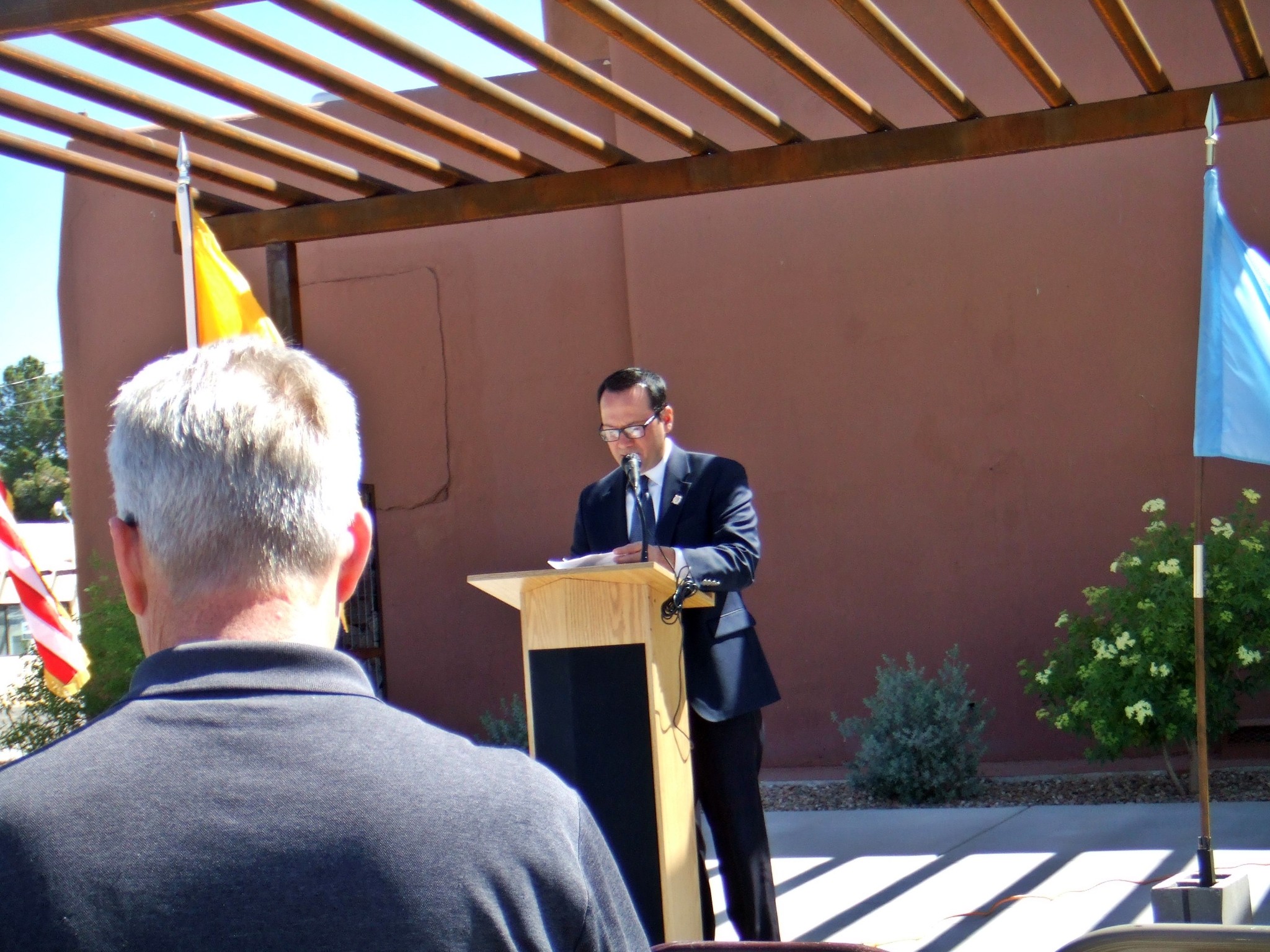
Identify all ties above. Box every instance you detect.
[629,476,656,546]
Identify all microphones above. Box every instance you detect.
[621,452,641,490]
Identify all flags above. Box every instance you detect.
[0,481,92,700]
[1192,165,1270,466]
[174,185,286,347]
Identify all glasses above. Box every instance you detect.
[598,407,660,442]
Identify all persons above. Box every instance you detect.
[568,367,782,942]
[0,334,653,952]
